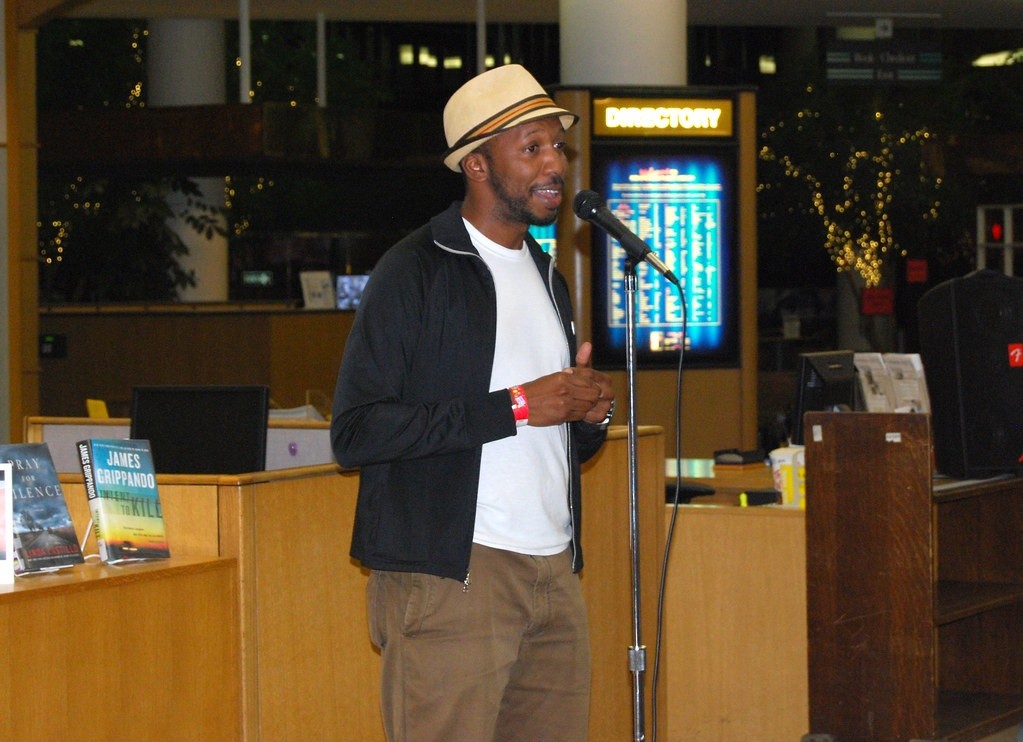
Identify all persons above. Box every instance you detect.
[329,62,615,742]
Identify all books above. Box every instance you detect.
[0,441,85,572]
[76,438,171,564]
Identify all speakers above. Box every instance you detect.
[918,269,1023,480]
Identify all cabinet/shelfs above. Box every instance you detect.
[23,414,665,741]
[804,411,1023,742]
[665,464,809,742]
[0,551,243,742]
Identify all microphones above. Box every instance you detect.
[572,190,677,284]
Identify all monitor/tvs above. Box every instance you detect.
[590,142,739,371]
[131,385,269,474]
[791,350,855,445]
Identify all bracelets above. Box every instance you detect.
[597,398,616,426]
[510,385,529,427]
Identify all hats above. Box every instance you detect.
[440,64,579,173]
